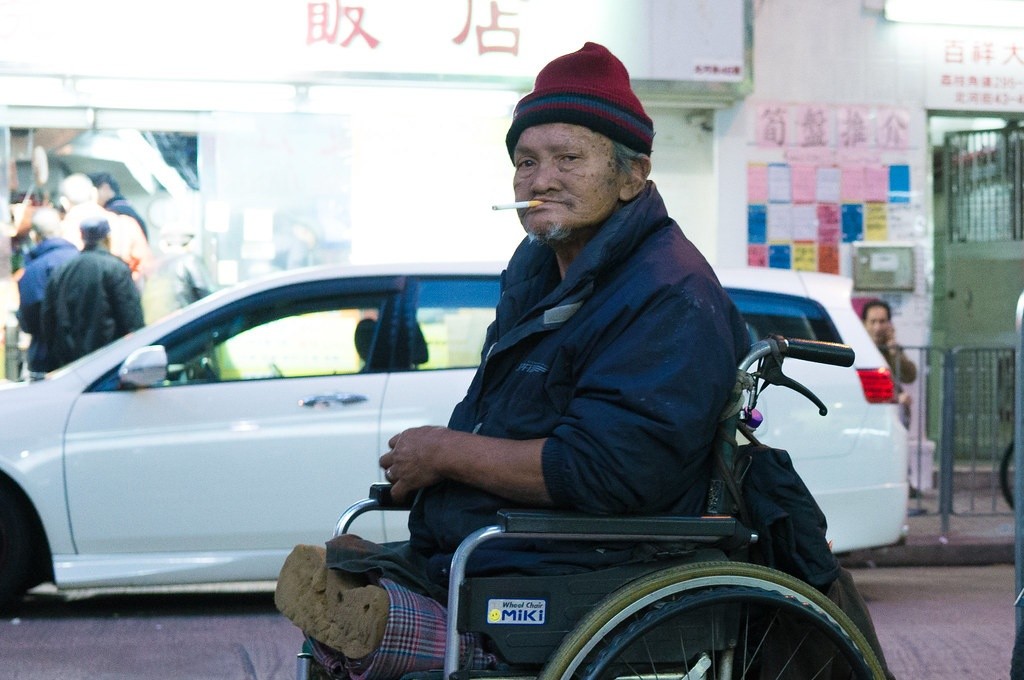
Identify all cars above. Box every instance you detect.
[0,263,909,609]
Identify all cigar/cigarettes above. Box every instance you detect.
[490,200,546,210]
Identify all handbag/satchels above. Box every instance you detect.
[731,423,898,680]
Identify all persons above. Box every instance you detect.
[377,43,744,596]
[858,299,927,518]
[3,156,150,395]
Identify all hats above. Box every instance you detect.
[505,42,653,165]
[80,217,111,242]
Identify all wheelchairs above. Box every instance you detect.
[298,323,893,680]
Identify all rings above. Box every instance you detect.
[386,466,396,482]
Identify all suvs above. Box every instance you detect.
[64,476,77,480]
